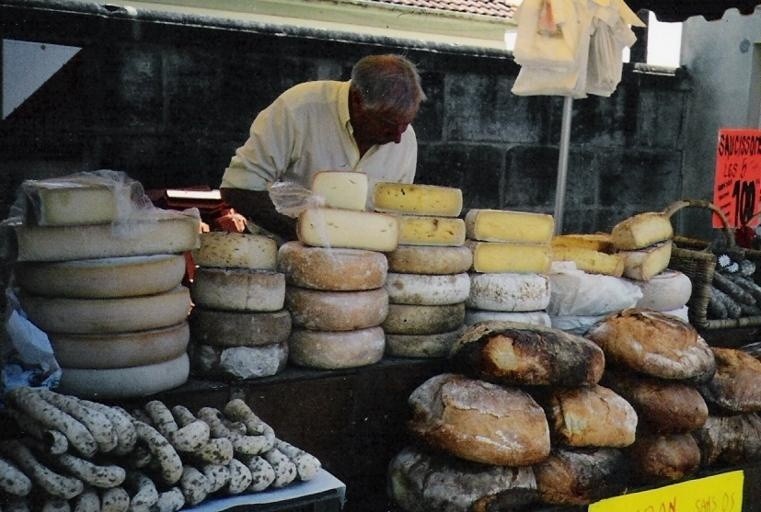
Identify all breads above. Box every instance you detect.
[1,171,760,399]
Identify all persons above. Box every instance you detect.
[220,52,428,253]
